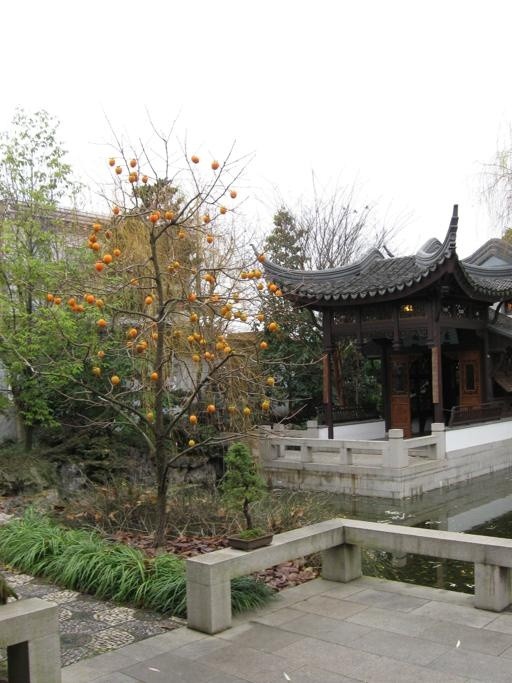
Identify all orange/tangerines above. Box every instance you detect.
[46,155,282,446]
